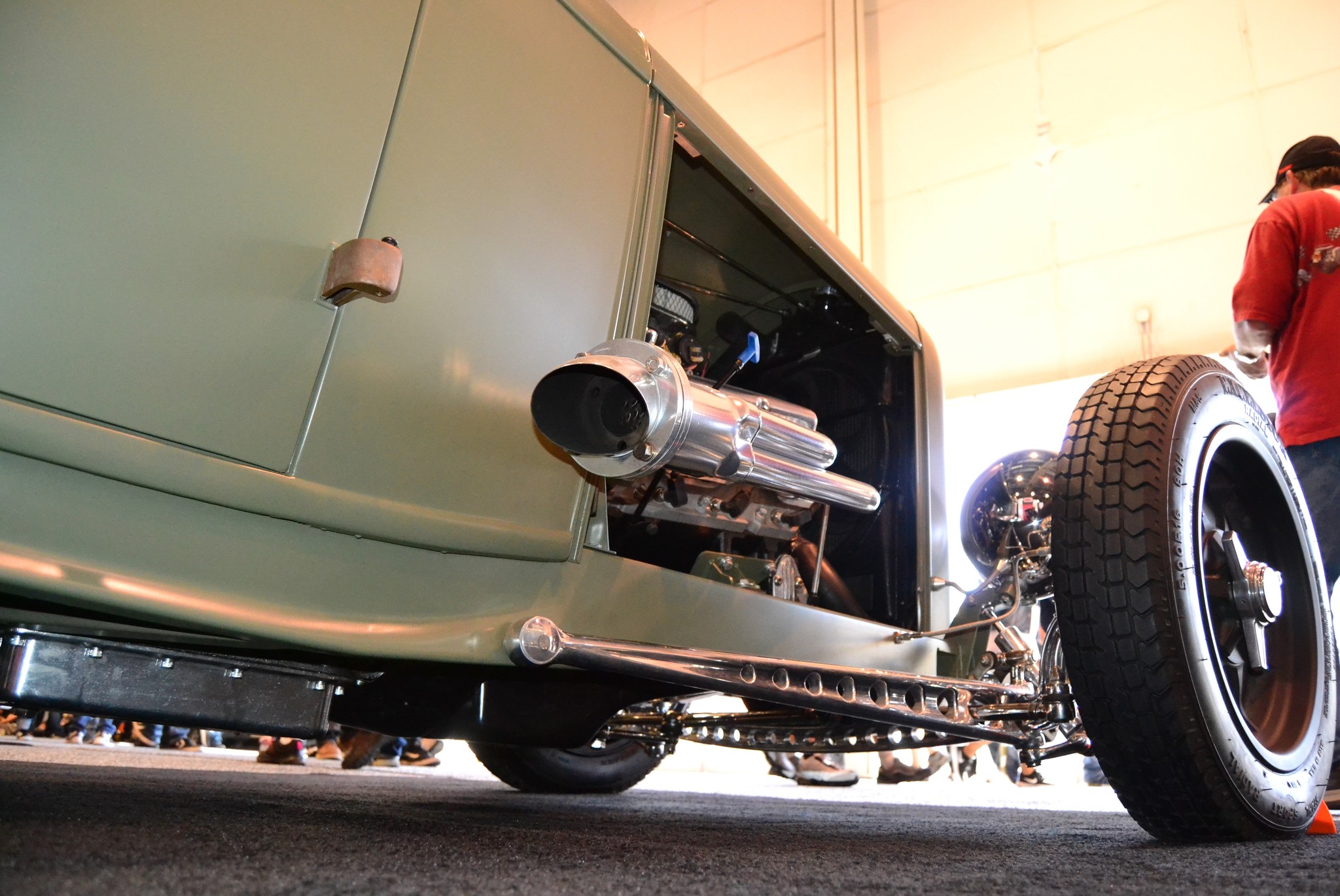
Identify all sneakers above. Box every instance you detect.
[797,756,858,787]
[876,758,933,784]
[1017,769,1053,786]
[927,751,948,775]
[958,748,977,781]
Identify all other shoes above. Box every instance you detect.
[0,709,444,770]
[1322,768,1340,810]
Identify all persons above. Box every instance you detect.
[1220,136,1340,809]
[0,704,448,771]
[760,718,1112,788]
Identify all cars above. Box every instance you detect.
[2,0,1340,839]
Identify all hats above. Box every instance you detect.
[1258,135,1340,205]
